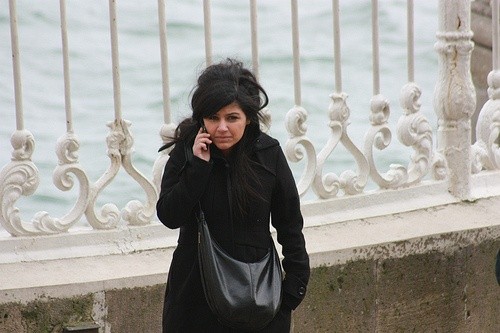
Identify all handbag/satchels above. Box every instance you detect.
[198,220,285,332]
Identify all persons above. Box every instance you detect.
[155,56,311,332]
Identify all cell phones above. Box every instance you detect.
[202,124,208,152]
